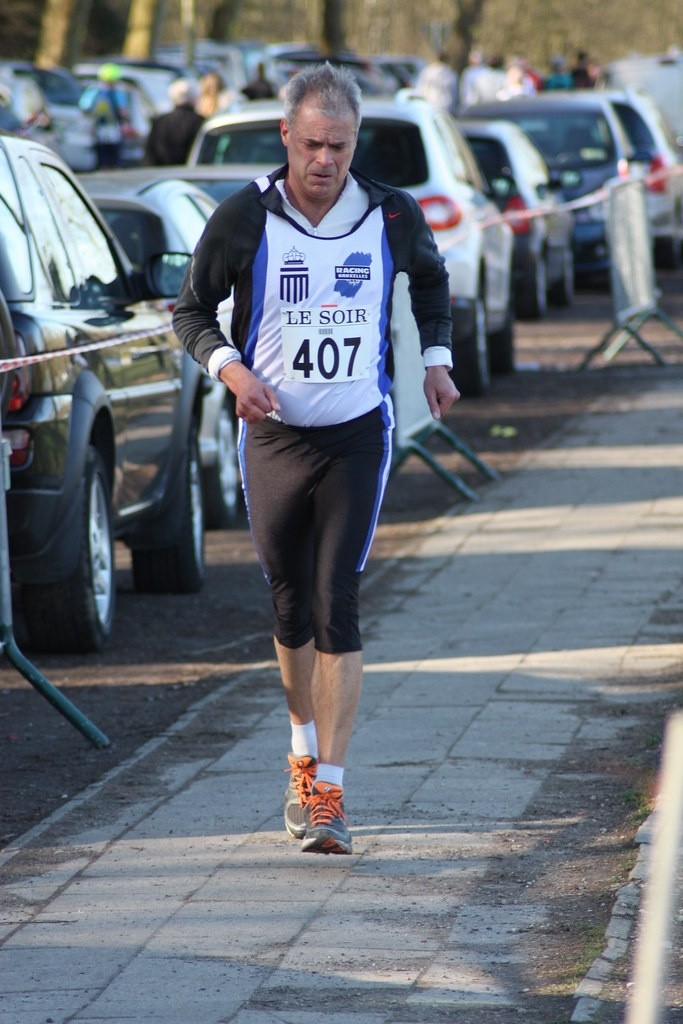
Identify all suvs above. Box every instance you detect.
[0,135,220,656]
[181,86,520,401]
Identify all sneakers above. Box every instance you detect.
[301,781,353,855]
[283,753,318,839]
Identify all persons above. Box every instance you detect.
[79,63,132,169]
[416,49,599,114]
[143,78,219,166]
[195,73,246,119]
[241,63,272,101]
[172,61,460,856]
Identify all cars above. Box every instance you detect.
[466,94,656,286]
[454,117,584,321]
[0,38,466,181]
[583,89,683,268]
[63,174,258,533]
